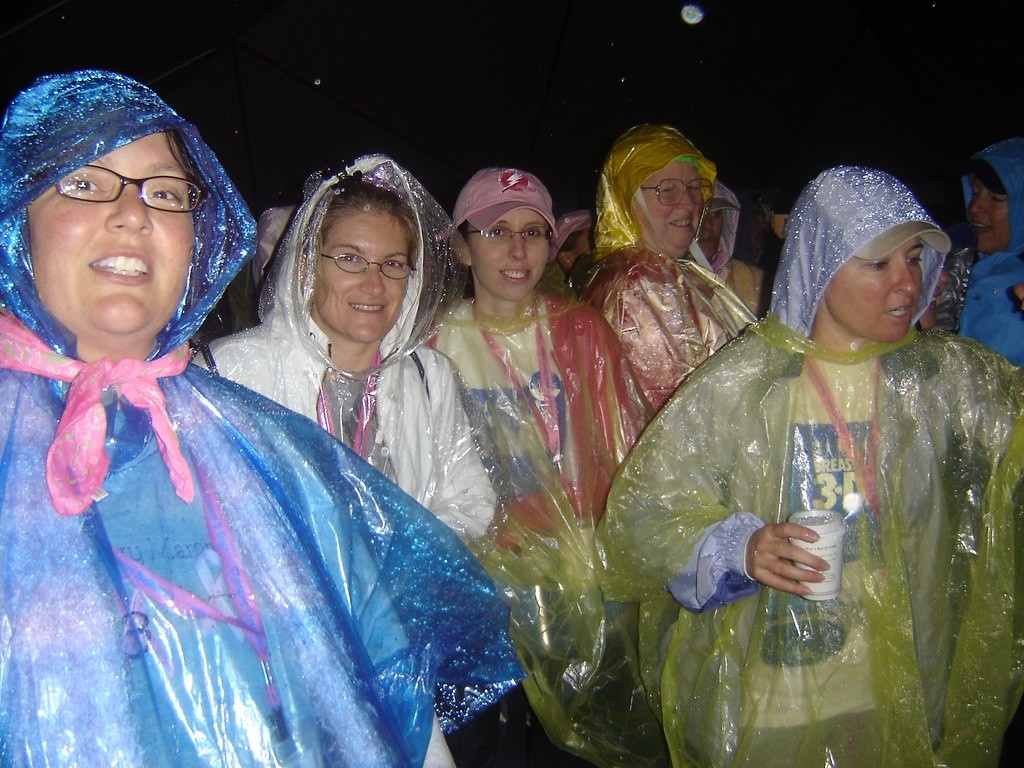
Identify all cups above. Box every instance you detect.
[786,510,846,602]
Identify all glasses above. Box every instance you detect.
[59,164,206,215]
[643,179,713,205]
[466,225,552,240]
[320,251,416,279]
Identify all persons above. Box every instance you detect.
[596,161,1024,767]
[2,65,457,768]
[189,161,500,545]
[937,138,1022,364]
[569,122,760,409]
[542,187,597,279]
[410,163,652,768]
[695,177,770,315]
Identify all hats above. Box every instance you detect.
[941,159,1008,194]
[452,169,560,236]
[853,221,951,259]
